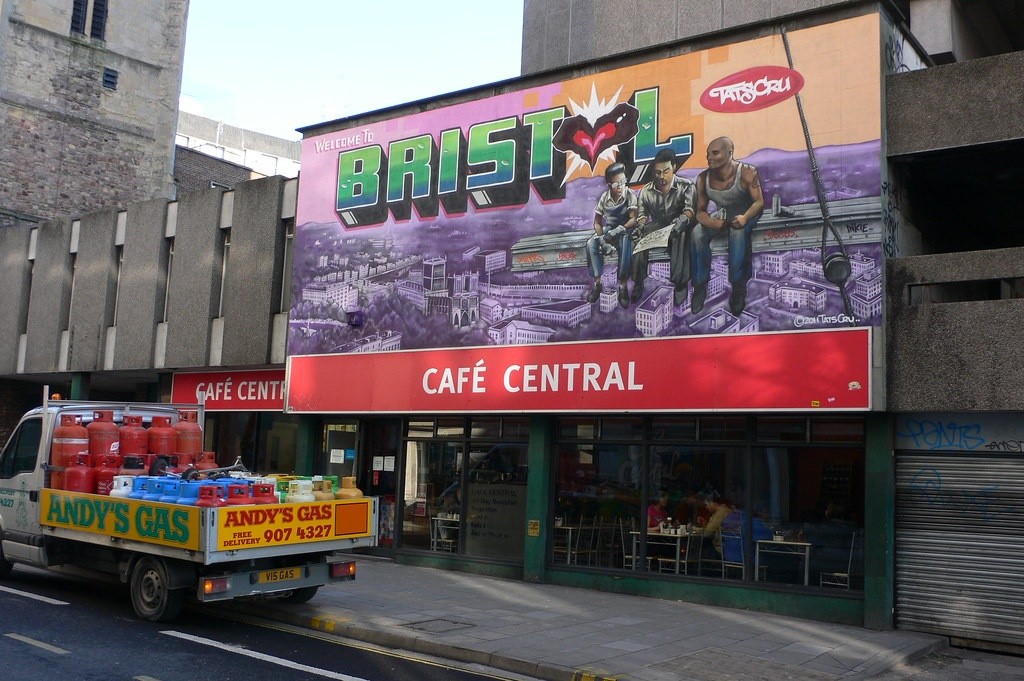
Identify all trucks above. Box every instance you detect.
[0,386,379,624]
[439,442,735,571]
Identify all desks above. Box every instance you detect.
[597,523,639,568]
[630,531,688,575]
[431,517,459,552]
[754,539,813,586]
[554,526,619,569]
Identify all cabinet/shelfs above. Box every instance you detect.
[816,461,855,524]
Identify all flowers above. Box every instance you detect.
[771,527,784,536]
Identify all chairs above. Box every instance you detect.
[553,512,768,582]
[818,532,856,590]
[428,505,458,552]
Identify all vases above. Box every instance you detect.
[772,534,784,542]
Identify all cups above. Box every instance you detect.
[446,514,459,520]
[665,523,689,534]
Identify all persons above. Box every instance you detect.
[647,490,733,560]
[436,494,456,539]
[797,505,849,572]
